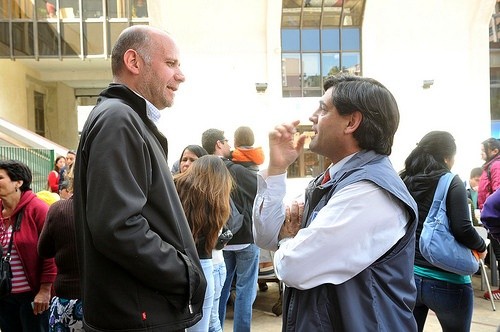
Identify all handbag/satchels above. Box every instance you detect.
[0,259,17,293]
[418,173,480,274]
[216,229,232,250]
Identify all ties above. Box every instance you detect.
[322,168,332,185]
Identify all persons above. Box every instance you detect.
[465,138,500,301]
[0,152,85,332]
[74,25,207,332]
[252,75,417,332]
[132,0,148,17]
[171,126,264,332]
[46,0,63,56]
[401,132,487,332]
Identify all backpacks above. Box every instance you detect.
[217,161,248,235]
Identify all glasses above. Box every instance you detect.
[220,138,229,144]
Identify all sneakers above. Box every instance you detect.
[485,287,500,302]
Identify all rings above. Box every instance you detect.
[285,219,290,222]
